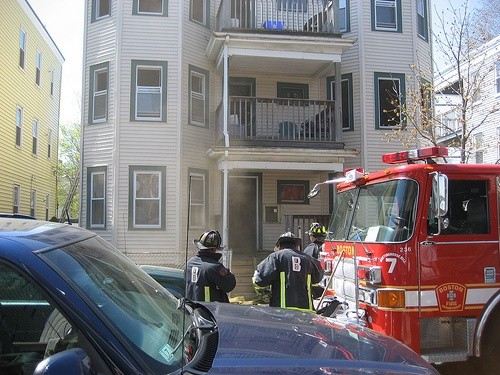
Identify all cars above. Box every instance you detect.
[0,213,441,375]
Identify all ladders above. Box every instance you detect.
[59,171,80,221]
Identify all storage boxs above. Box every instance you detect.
[262,21,284,30]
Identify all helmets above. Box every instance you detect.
[276,232,302,248]
[306,222,326,234]
[193,230,224,250]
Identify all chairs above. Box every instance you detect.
[0,304,40,375]
[465,197,488,233]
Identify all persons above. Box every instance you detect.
[303,222,327,259]
[252,232,324,313]
[185,230,236,304]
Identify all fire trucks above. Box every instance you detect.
[307,146,500,375]
